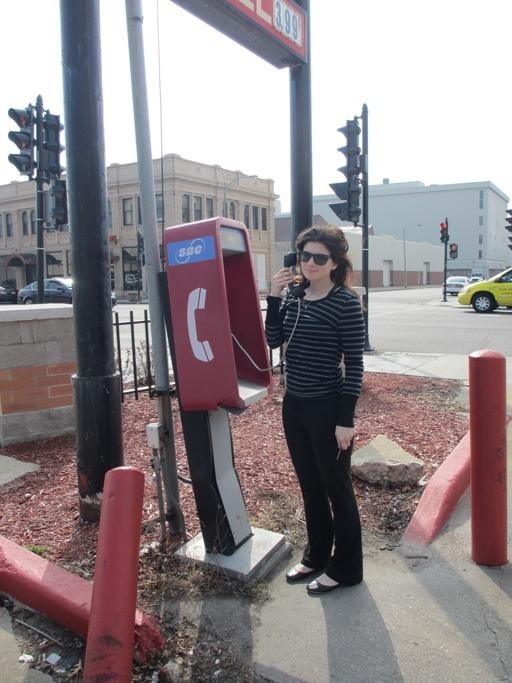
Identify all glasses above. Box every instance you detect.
[300,250,332,265]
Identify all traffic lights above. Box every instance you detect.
[328,118,360,225]
[506,236,512,250]
[439,221,448,242]
[503,207,512,232]
[449,243,458,257]
[43,110,66,182]
[5,102,35,175]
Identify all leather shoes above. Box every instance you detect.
[286,563,319,580]
[306,577,339,593]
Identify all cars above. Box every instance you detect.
[441,275,471,295]
[16,275,117,306]
[469,271,483,283]
[125,275,140,287]
[455,264,512,314]
[1,281,18,302]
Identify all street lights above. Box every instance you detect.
[402,222,425,288]
[222,171,259,218]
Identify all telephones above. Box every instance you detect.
[284,252,306,299]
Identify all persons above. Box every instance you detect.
[265,225,364,595]
